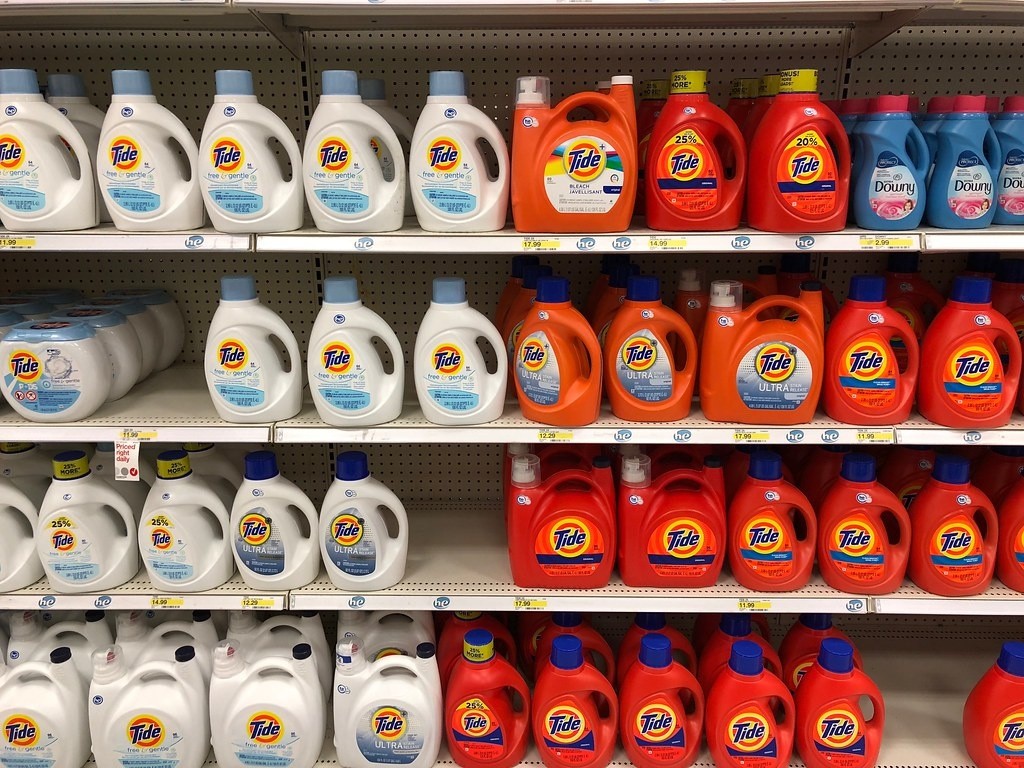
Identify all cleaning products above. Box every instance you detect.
[0,63,1023,768]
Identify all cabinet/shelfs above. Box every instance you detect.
[0,0,1024,768]
